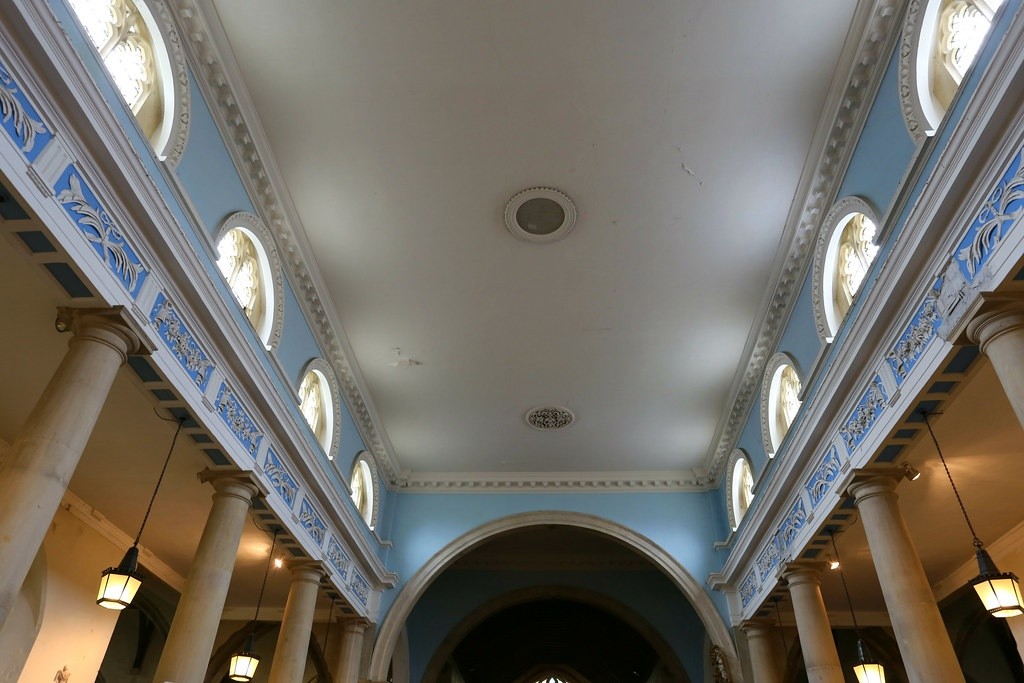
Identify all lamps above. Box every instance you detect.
[229,525,287,683]
[916,406,1024,618]
[827,527,886,683]
[901,462,920,481]
[96,415,189,612]
[503,187,578,245]
